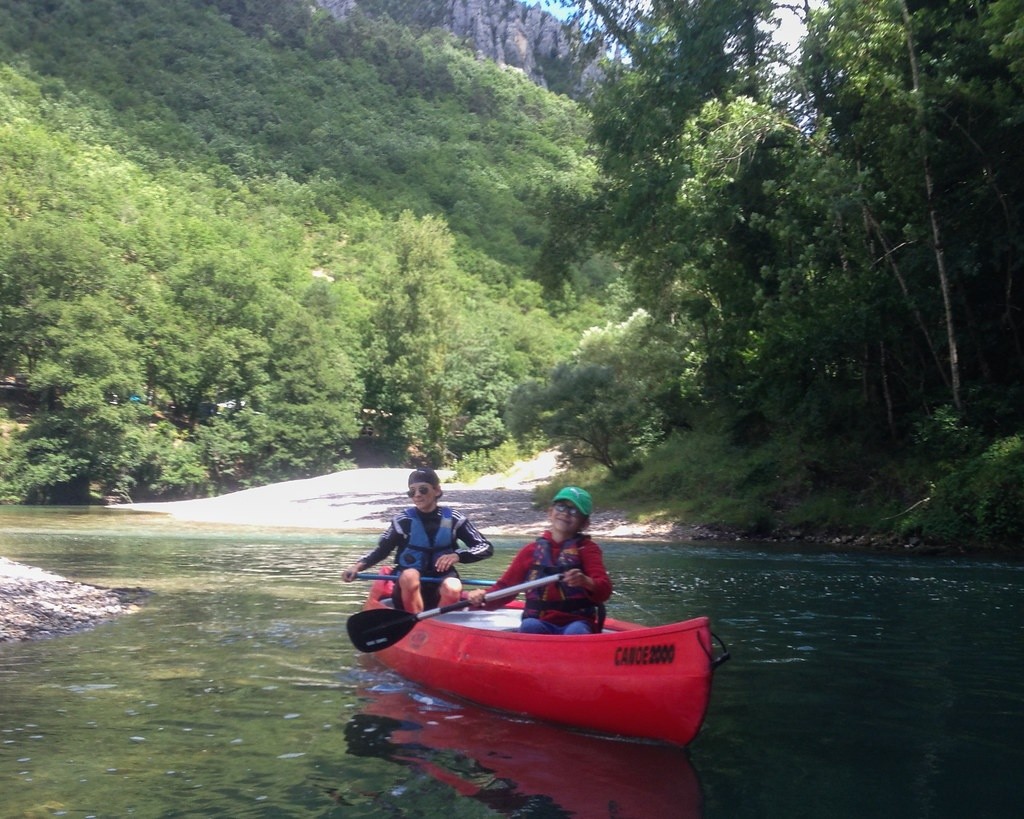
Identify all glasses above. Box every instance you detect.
[553,502,582,517]
[407,486,433,498]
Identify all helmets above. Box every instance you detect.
[408,468,439,486]
[550,486,592,518]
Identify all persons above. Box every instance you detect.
[342,466,494,614]
[467,486,613,636]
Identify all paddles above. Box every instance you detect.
[345,571,566,655]
[340,566,505,590]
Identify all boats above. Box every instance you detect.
[361,567,729,745]
